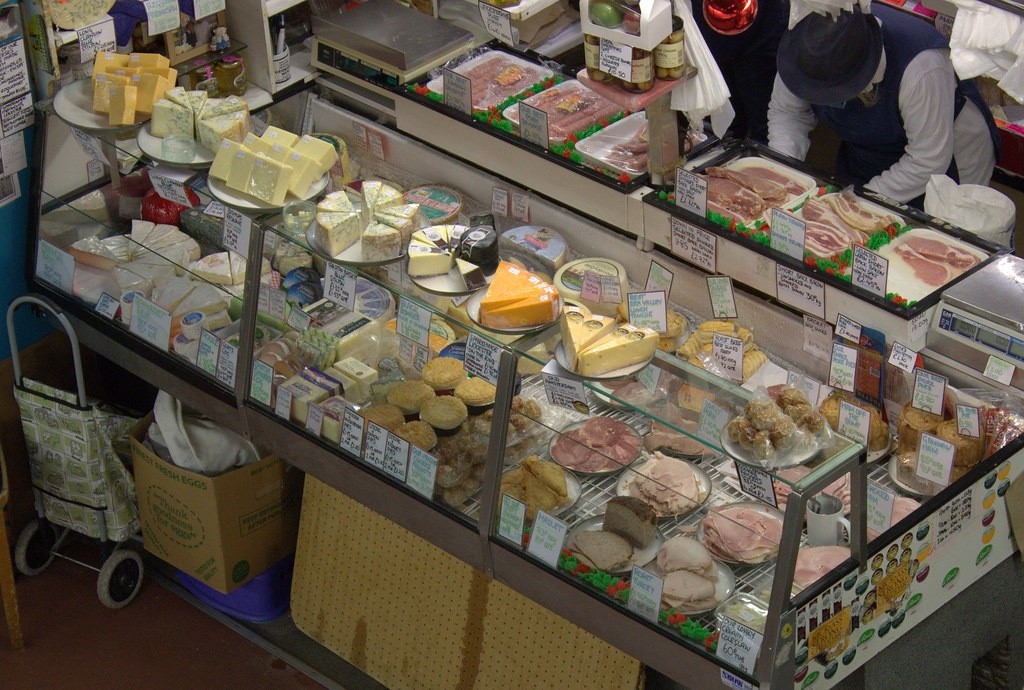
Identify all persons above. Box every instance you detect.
[766,0,995,209]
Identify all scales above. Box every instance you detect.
[309,1,478,91]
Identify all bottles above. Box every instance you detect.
[176,52,248,98]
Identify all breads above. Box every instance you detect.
[727,388,987,490]
[617,302,824,462]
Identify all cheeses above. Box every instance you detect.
[65,51,659,438]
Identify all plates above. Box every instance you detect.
[305,203,430,264]
[466,284,564,332]
[889,453,932,497]
[776,502,851,519]
[867,497,922,544]
[206,169,330,210]
[590,381,667,407]
[556,339,655,378]
[52,78,152,132]
[791,546,851,591]
[499,417,782,614]
[136,121,215,164]
[866,432,893,463]
[408,257,502,291]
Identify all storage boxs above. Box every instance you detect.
[285,469,651,690]
[126,401,298,597]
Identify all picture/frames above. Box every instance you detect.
[166,9,228,68]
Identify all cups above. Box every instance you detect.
[272,43,291,84]
[161,136,197,162]
[401,387,495,453]
[283,201,317,233]
[806,494,851,547]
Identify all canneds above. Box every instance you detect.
[189,62,221,99]
[584,15,686,94]
[215,55,246,96]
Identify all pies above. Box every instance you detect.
[366,358,498,455]
